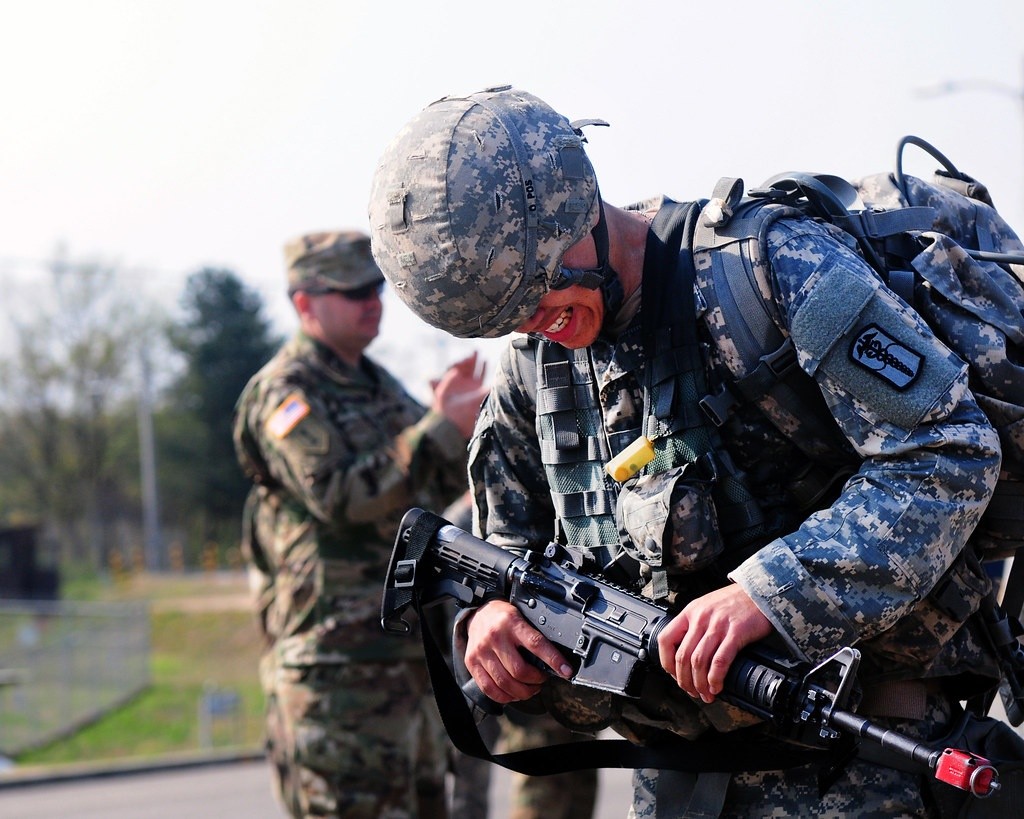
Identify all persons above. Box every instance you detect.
[368,85,1024,819]
[232,231,601,819]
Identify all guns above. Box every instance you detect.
[380,507,1005,801]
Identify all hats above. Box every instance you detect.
[286,232,385,298]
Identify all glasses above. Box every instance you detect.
[322,278,386,301]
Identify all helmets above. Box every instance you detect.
[370,85,599,342]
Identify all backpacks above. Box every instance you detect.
[692,134,1024,555]
